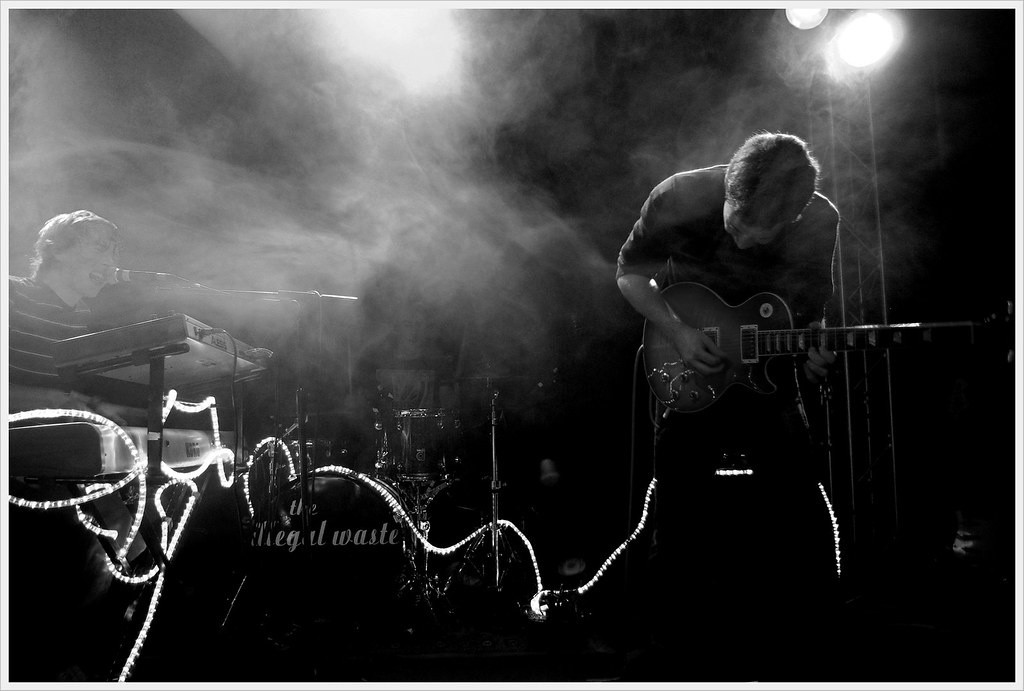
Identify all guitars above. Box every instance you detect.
[640,280,1015,415]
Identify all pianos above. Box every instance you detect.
[8,419,252,480]
[46,311,284,398]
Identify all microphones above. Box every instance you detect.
[106,266,174,285]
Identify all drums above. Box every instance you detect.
[281,436,336,475]
[371,407,467,484]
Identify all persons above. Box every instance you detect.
[10,205,152,601]
[578,133,866,653]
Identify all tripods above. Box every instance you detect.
[398,388,525,619]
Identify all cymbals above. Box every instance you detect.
[442,357,532,384]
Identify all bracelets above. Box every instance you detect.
[87,396,101,411]
